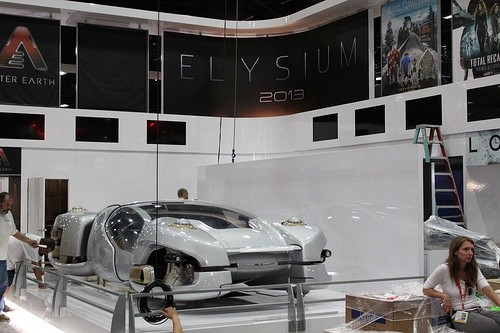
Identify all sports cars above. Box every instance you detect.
[48,199,331,304]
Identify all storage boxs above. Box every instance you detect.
[478,279,500,295]
[431,298,450,333]
[345,295,433,333]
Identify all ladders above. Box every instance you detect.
[413,125,468,229]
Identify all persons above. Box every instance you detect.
[0,192,39,321]
[422,236,500,333]
[160,306,183,333]
[176,188,189,199]
[474,0,489,56]
[6,232,56,297]
[490,3,500,51]
